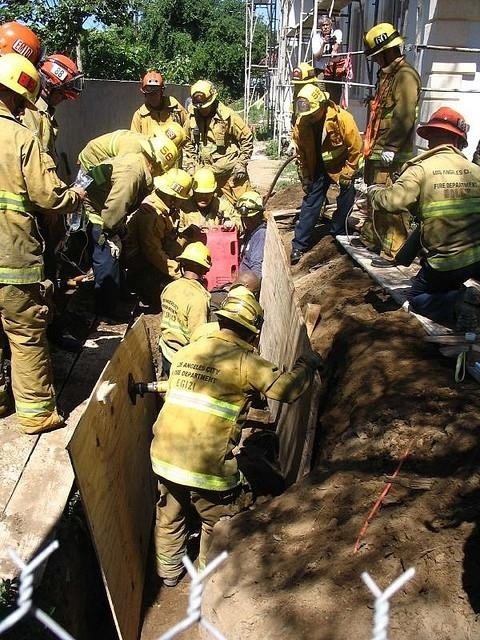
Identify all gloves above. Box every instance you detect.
[230,162,248,182]
[295,347,325,375]
[380,150,395,168]
[104,231,124,261]
[301,182,311,195]
[222,220,236,232]
[338,172,353,191]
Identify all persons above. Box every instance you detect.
[289,84,363,264]
[25,55,84,230]
[311,15,344,105]
[351,21,422,269]
[75,70,268,343]
[0,52,89,435]
[158,241,213,379]
[355,106,480,335]
[290,61,327,131]
[148,293,320,587]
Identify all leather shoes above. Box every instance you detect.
[350,238,365,247]
[371,255,398,268]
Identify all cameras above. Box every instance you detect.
[328,36,336,45]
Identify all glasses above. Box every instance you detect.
[50,73,85,93]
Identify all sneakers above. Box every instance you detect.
[162,575,180,588]
[289,247,302,265]
[452,286,480,337]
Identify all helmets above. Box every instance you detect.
[290,61,331,117]
[174,240,212,272]
[138,70,166,95]
[212,283,264,335]
[190,79,219,110]
[415,106,471,149]
[37,53,86,102]
[137,120,218,201]
[362,22,407,59]
[233,190,264,218]
[1,18,42,113]
